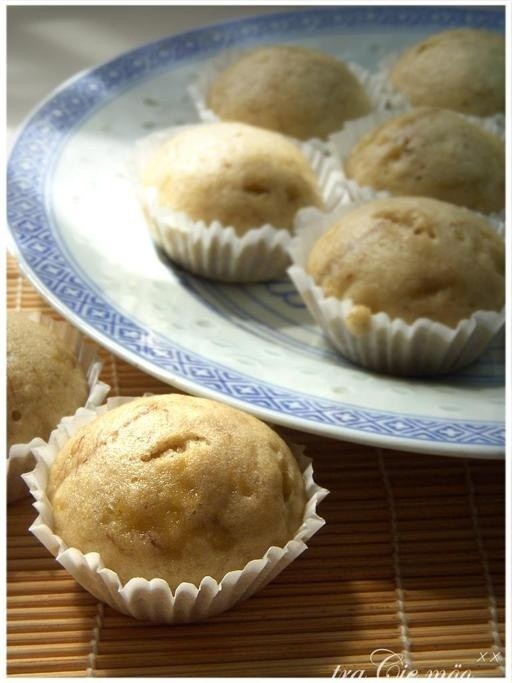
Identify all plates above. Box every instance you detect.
[7,6,504,461]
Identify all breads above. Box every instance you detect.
[138,29,505,340]
[45,393,306,597]
